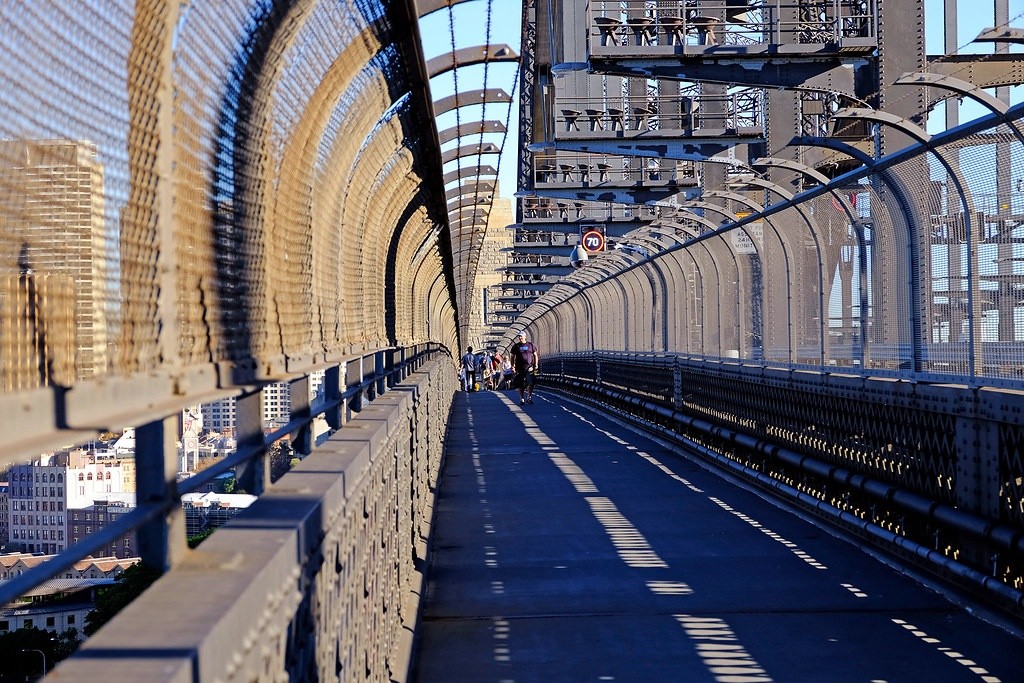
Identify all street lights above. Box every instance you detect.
[22,648,46,679]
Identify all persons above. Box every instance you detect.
[462,346,477,393]
[469,351,518,391]
[511,331,539,406]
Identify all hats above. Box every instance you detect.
[517,330,526,336]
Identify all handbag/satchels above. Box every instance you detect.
[474,382,479,392]
[483,370,491,379]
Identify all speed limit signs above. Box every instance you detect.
[581,230,604,253]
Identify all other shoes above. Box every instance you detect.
[520,401,525,406]
[526,399,534,405]
